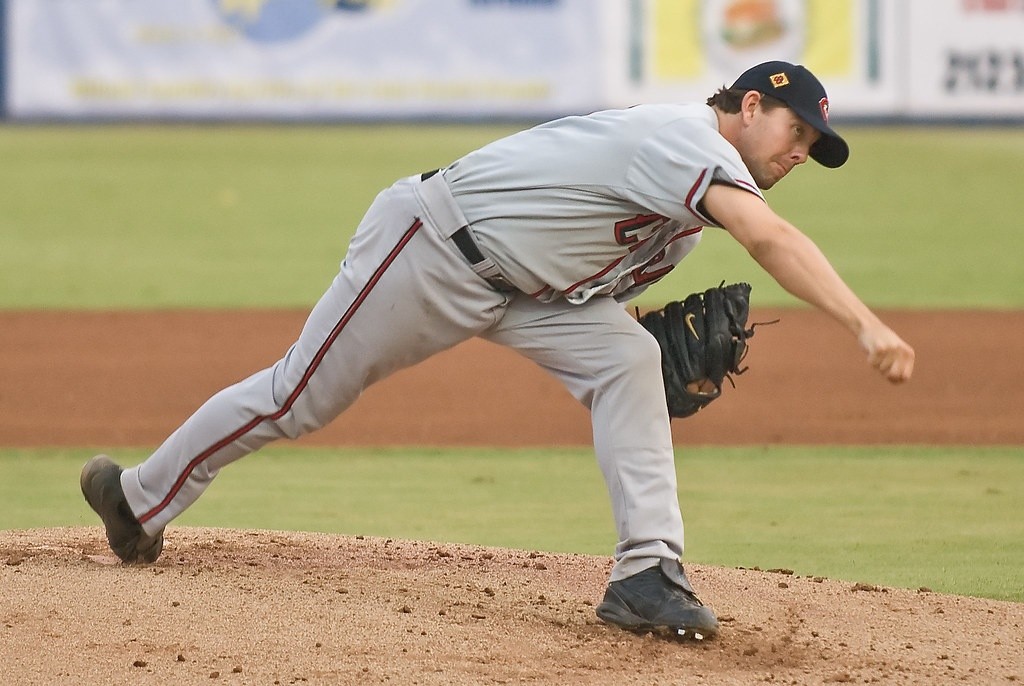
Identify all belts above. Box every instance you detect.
[423,168,521,296]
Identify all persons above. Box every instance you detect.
[80,61,917,640]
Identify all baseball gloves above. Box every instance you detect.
[634,280,755,419]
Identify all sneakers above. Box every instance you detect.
[78,454,166,564]
[595,561,719,641]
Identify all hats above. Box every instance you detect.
[728,61,851,169]
